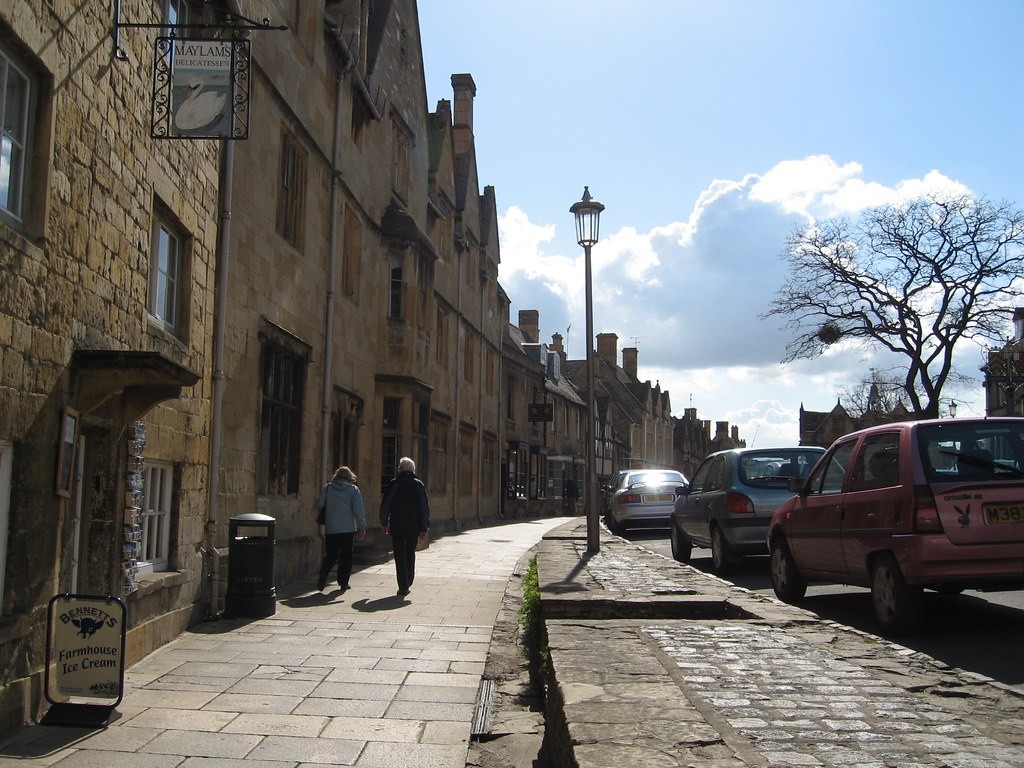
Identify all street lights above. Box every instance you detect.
[569,186,605,550]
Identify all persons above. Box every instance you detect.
[566,476,581,517]
[314,465,368,592]
[379,456,432,596]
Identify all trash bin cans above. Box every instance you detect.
[224,513,277,617]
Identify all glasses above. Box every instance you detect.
[397,467,400,472]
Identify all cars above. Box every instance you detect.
[764,420,1024,628]
[671,446,847,577]
[602,470,690,534]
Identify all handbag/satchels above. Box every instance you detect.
[316,484,329,525]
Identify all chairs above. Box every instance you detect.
[868,454,897,476]
[956,450,992,473]
[777,463,801,475]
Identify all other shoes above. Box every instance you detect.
[397,586,410,595]
[318,584,324,591]
[341,583,351,590]
